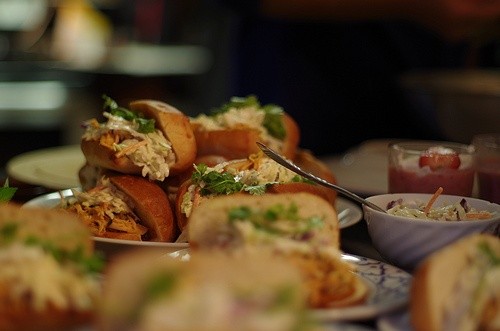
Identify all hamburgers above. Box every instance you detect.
[0,94,369,331]
[408,232,500,331]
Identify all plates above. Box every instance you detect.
[169,249,416,323]
[5,144,86,189]
[377,304,411,330]
[20,189,363,254]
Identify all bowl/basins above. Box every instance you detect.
[363,192,500,269]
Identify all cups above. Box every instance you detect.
[388,140,478,198]
[473,134,500,204]
